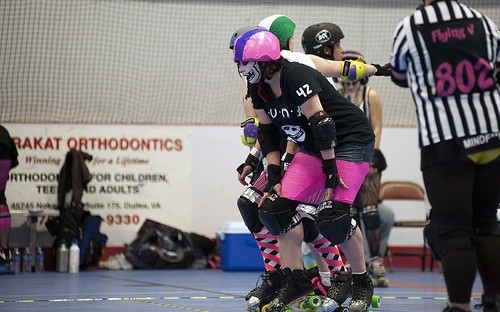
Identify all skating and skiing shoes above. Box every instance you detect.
[365,257,389,287]
[262,267,322,312]
[303,263,326,296]
[247,268,288,312]
[245,266,292,300]
[322,271,353,312]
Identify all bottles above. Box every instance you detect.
[22,247,33,275]
[57,240,69,272]
[10,248,20,273]
[35,247,44,274]
[69,240,79,274]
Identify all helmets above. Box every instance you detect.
[333,51,368,84]
[229,26,267,50]
[257,14,296,50]
[233,29,281,62]
[300,22,344,58]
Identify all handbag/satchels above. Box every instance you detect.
[132,230,197,269]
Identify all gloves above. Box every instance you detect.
[371,62,392,76]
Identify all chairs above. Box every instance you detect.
[378,181,436,272]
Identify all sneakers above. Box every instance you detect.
[349,271,381,312]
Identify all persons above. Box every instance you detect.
[230,15,394,312]
[389,0,500,312]
[234,30,382,312]
[0,126,18,274]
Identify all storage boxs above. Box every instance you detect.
[215,221,265,273]
